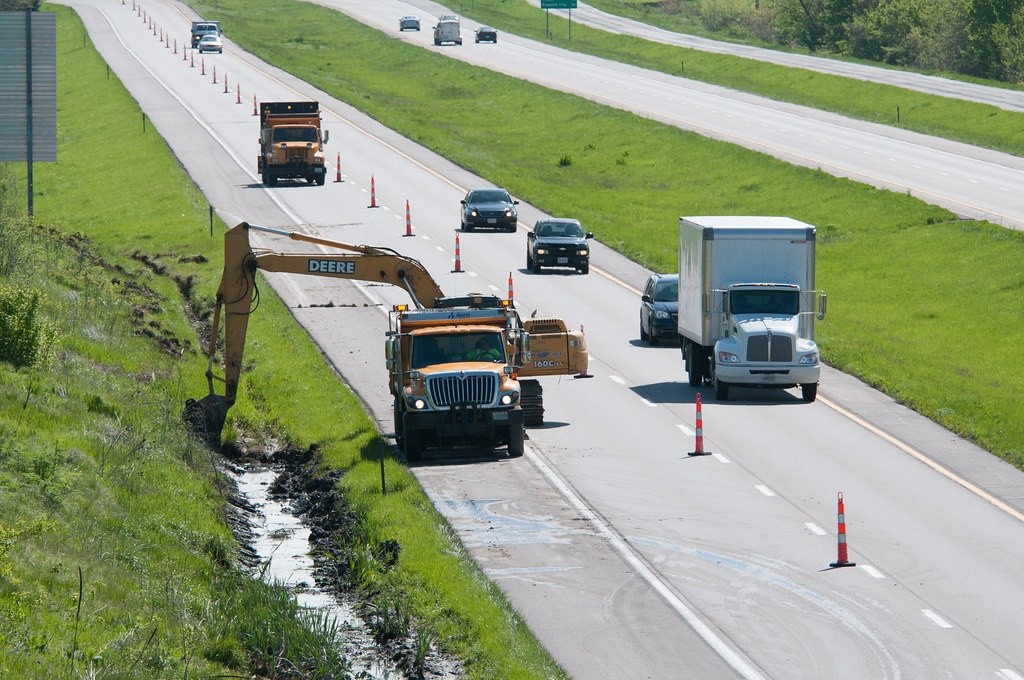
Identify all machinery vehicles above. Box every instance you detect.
[180,223,593,461]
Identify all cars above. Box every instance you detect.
[399,15,420,30]
[459,188,517,234]
[190,19,221,52]
[637,272,678,344]
[473,25,497,43]
[525,218,593,274]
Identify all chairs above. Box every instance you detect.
[543,225,552,234]
[566,226,576,235]
[302,130,312,138]
[418,336,443,363]
[275,130,289,139]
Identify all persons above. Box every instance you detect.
[467,337,501,361]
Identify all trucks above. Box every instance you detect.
[256,100,327,185]
[679,215,831,406]
[433,13,462,47]
